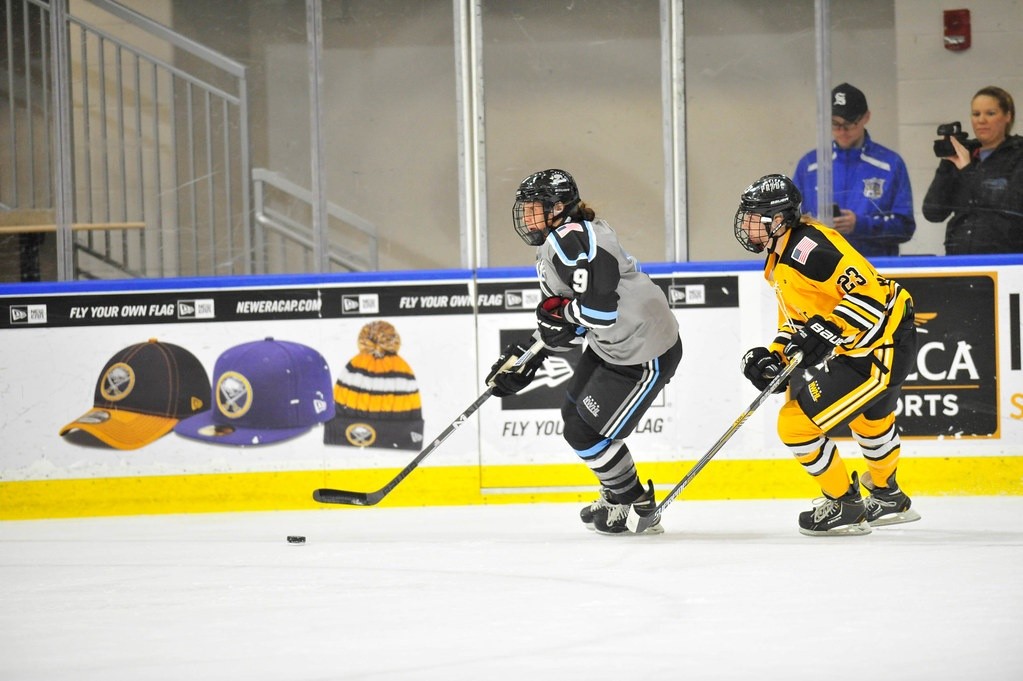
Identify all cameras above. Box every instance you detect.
[934,121,969,157]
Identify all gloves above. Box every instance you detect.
[485,337,554,397]
[783,315,844,369]
[740,347,791,394]
[534,293,587,348]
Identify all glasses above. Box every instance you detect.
[832,113,865,131]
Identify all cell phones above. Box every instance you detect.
[832,203,841,219]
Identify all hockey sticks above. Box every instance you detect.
[623,350,804,535]
[310,338,545,507]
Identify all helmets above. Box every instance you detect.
[512,169,581,246]
[733,174,801,254]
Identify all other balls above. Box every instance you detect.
[286,535,306,544]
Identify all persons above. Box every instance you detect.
[734,173,920,537]
[792,82,916,258]
[922,85,1023,255]
[487,168,683,537]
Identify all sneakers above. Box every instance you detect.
[593,480,664,535]
[798,471,872,536]
[580,488,613,531]
[861,471,921,527]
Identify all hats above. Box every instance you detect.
[323,321,424,450]
[59,337,211,450]
[174,336,335,445]
[831,83,868,122]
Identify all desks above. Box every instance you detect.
[0,208,146,282]
